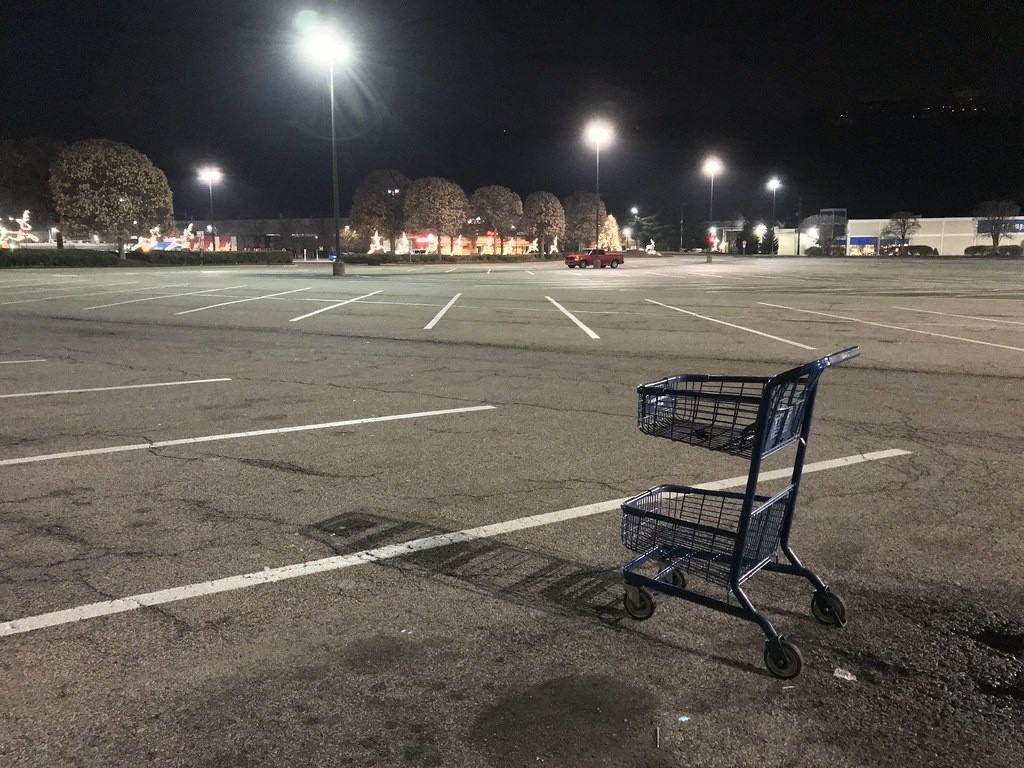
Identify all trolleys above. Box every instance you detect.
[620,346,863,680]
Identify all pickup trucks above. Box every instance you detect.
[565,247,624,269]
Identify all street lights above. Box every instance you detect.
[317,33,345,277]
[592,129,605,268]
[770,181,778,257]
[205,172,216,251]
[707,162,715,262]
[632,208,639,252]
